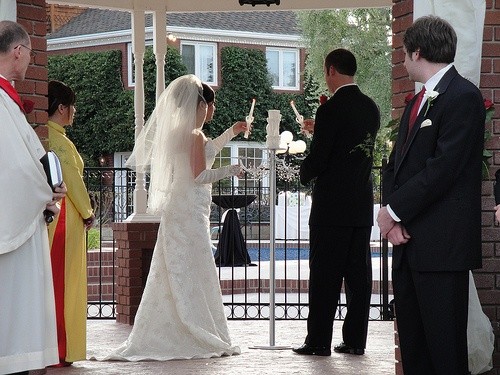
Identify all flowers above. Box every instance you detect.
[424,90,440,118]
[319,94,328,105]
[22,97,40,129]
[483,97,495,111]
[404,92,414,105]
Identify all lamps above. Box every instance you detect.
[269,130,306,159]
[166,33,177,41]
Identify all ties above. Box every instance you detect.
[408,86,426,136]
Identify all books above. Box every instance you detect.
[40,149,64,193]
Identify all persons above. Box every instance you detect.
[291,48,380,358]
[492,168,500,224]
[90,73,252,363]
[47,80,95,368]
[0,18,68,375]
[376,13,486,375]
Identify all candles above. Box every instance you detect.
[243,98,256,139]
[266,104,282,149]
[290,100,312,138]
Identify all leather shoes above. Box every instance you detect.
[335,341,364,355]
[292,343,331,356]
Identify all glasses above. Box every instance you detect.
[14,44,37,57]
